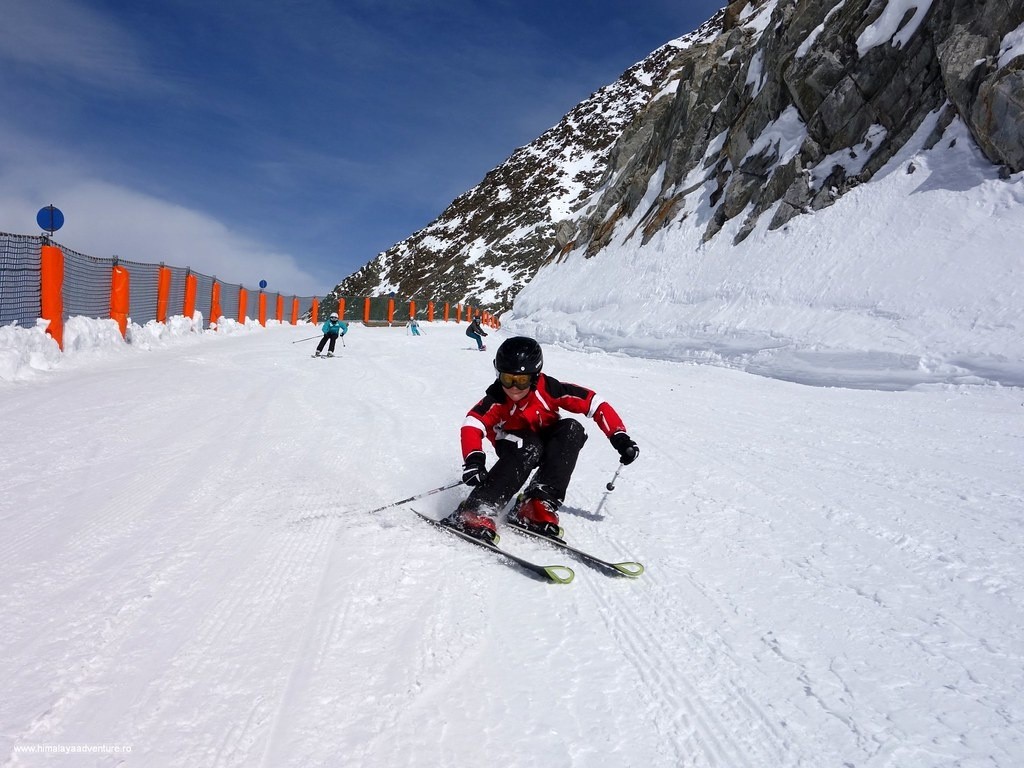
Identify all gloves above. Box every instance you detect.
[481,332,488,337]
[611,431,639,465]
[406,325,408,328]
[340,333,344,337]
[462,451,488,487]
[417,324,419,327]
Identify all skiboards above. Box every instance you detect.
[411,505,644,586]
[311,350,339,360]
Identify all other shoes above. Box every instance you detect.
[316,350,320,357]
[327,351,334,357]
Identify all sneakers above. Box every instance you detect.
[441,499,499,546]
[413,334,420,336]
[506,492,564,539]
[479,345,486,351]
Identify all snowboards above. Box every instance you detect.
[478,345,486,352]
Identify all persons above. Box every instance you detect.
[466,315,488,351]
[315,313,348,357]
[406,317,420,336]
[440,336,640,545]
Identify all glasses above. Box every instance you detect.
[330,316,337,319]
[500,373,530,391]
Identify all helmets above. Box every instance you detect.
[409,317,414,321]
[493,336,543,382]
[476,319,480,323]
[330,313,339,320]
